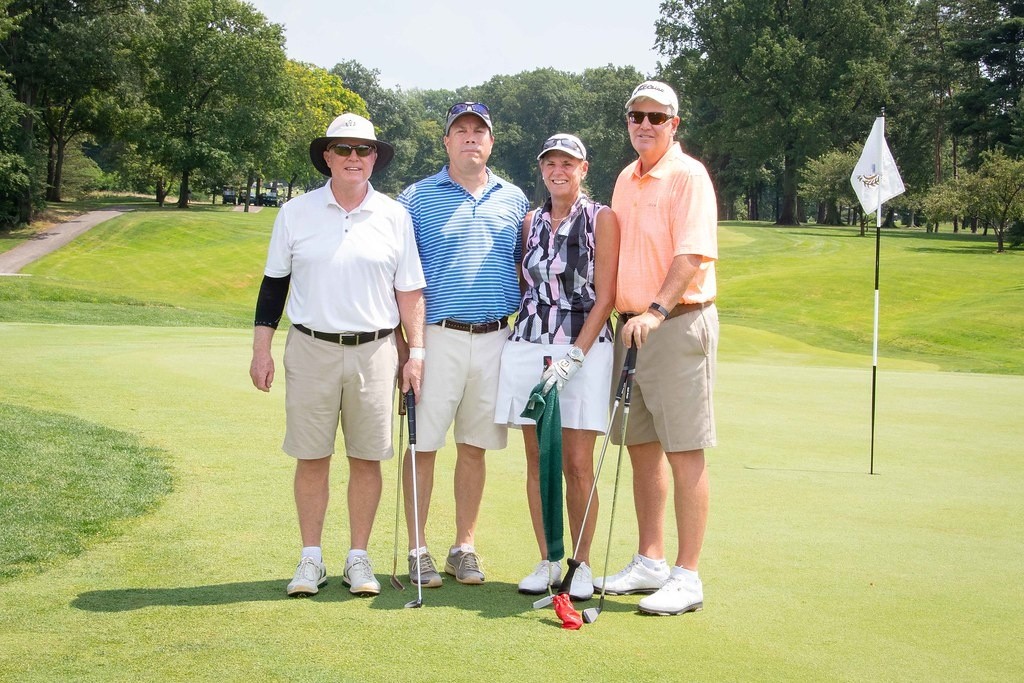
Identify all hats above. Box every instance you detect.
[445,105,494,140]
[309,116,395,177]
[537,135,586,162]
[625,81,679,114]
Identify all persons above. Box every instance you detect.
[249,112,428,599]
[593,81,720,614]
[492,134,620,599]
[394,100,531,587]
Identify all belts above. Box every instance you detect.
[293,323,394,348]
[619,300,713,324]
[438,317,509,335]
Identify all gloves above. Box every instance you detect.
[540,347,585,397]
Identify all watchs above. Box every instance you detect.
[567,347,585,362]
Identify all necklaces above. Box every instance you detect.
[551,215,562,221]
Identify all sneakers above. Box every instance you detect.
[518,559,563,593]
[342,558,380,594]
[572,560,594,601]
[408,552,442,588]
[594,555,670,596]
[285,554,327,596]
[637,572,704,616]
[444,547,486,585]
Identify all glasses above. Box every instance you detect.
[540,139,585,158]
[327,144,376,156]
[445,102,491,124]
[628,112,675,125]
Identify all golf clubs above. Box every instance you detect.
[581,335,639,624]
[531,355,558,609]
[404,387,424,609]
[555,346,632,599]
[388,374,406,594]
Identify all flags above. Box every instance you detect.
[851,116,907,216]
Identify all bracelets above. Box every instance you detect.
[410,346,426,360]
[650,302,669,319]
